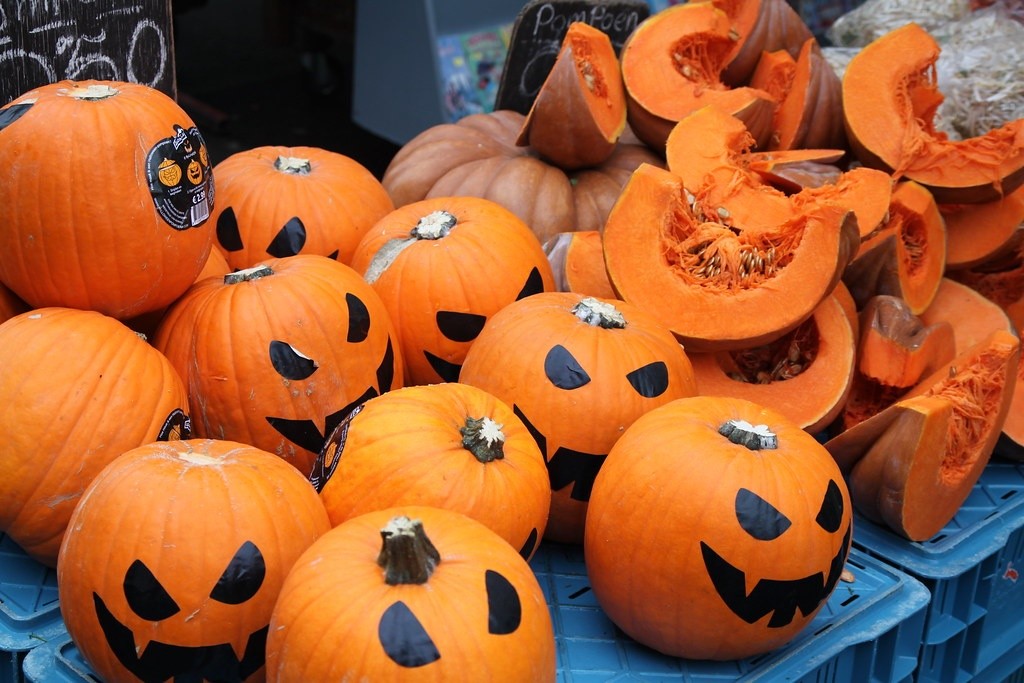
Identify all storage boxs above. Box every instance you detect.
[0,453,1024,683]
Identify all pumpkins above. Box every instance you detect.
[1,1,1024,683]
